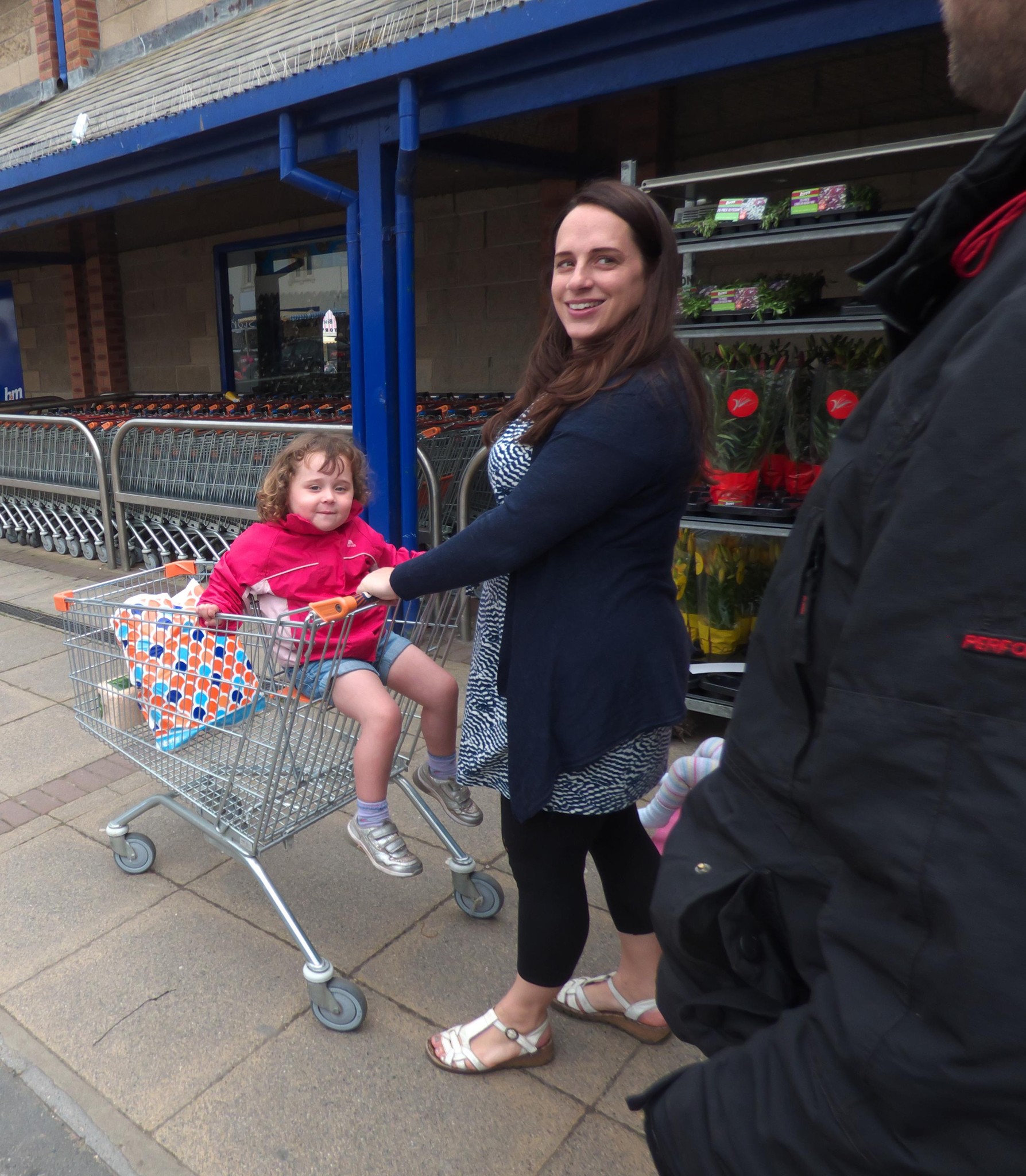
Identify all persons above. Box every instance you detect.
[634,0,1026,1176]
[193,432,483,878]
[359,175,707,1072]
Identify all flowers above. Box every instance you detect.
[692,336,887,471]
[672,528,783,629]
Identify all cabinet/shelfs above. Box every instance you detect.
[620,126,1003,720]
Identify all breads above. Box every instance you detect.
[98,674,146,730]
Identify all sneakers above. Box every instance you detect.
[345,813,422,877]
[412,761,483,827]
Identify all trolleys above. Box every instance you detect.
[0,392,509,596]
[51,558,506,1031]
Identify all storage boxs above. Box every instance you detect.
[98,673,146,731]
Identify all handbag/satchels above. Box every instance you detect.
[109,576,265,750]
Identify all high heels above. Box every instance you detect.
[552,969,670,1045]
[423,1006,552,1073]
